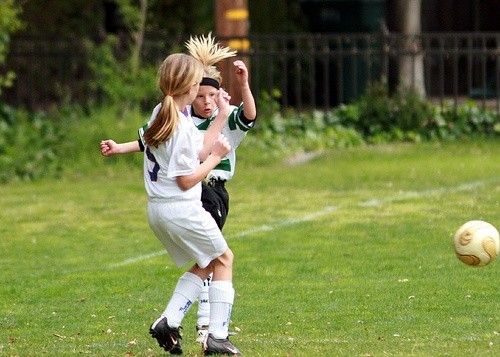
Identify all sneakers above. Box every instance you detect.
[203,332,241,354]
[149,316,184,355]
[195,324,208,342]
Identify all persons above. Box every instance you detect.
[356,0,390,97]
[99,31,256,345]
[143,53,243,357]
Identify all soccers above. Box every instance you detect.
[453,220,500,268]
